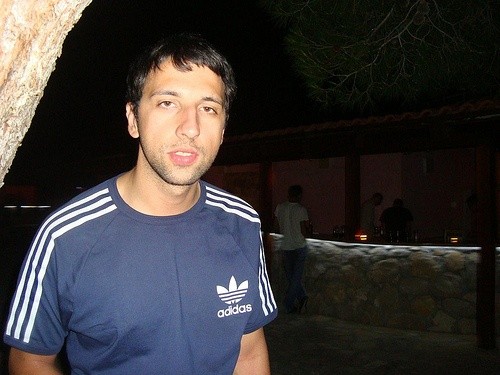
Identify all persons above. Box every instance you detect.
[360,193,384,241]
[3,36,278,375]
[380,198,414,241]
[274,185,309,315]
